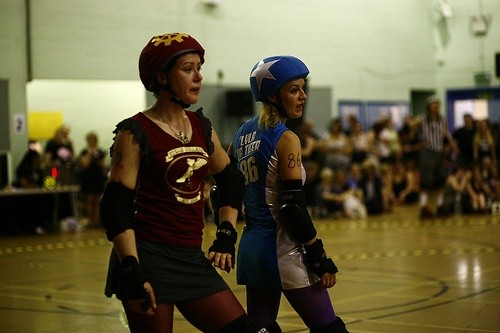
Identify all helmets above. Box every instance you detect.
[251,55,310,101]
[139,32,206,92]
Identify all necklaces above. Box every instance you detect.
[152,103,189,144]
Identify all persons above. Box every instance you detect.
[227,56,349,332]
[98,32,260,333]
[293,96,500,219]
[17,123,107,230]
